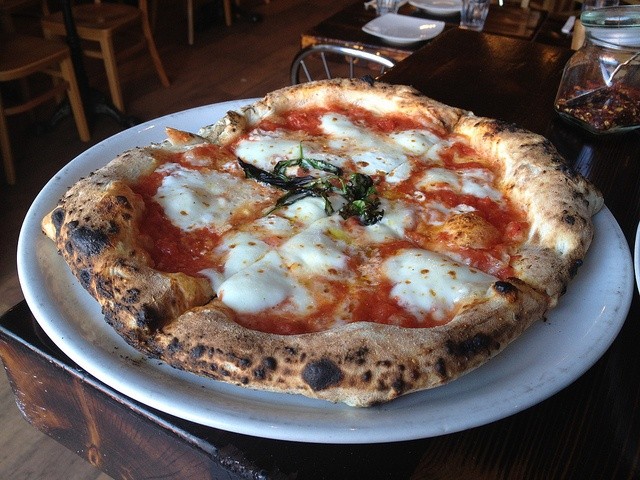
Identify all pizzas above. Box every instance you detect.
[40,74,605,410]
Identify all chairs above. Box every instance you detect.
[1,31,91,187]
[39,0,171,114]
[288,46,399,88]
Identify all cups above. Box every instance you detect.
[376,0,397,16]
[459,0,489,31]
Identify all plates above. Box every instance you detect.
[16,97,634,444]
[362,14,446,45]
[406,1,470,16]
